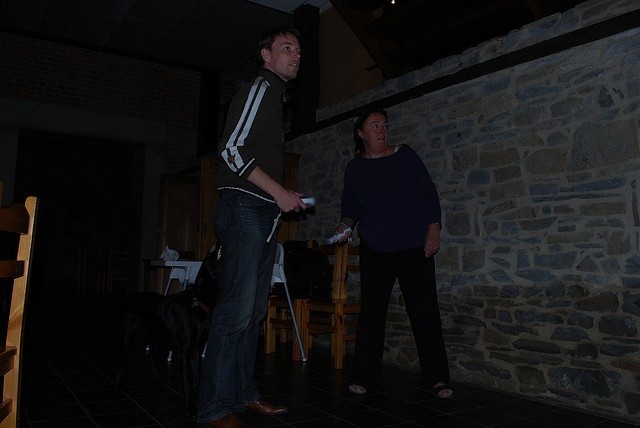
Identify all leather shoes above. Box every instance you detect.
[206,412,251,426]
[235,395,291,415]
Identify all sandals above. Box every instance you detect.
[349,379,372,395]
[431,384,453,398]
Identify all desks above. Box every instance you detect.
[148,243,305,362]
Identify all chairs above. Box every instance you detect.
[264,240,301,360]
[0,197,42,428]
[302,240,361,369]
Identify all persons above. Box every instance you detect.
[332,107,454,400]
[192,26,308,426]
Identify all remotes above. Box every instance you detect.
[300,195,316,207]
[326,227,352,246]
